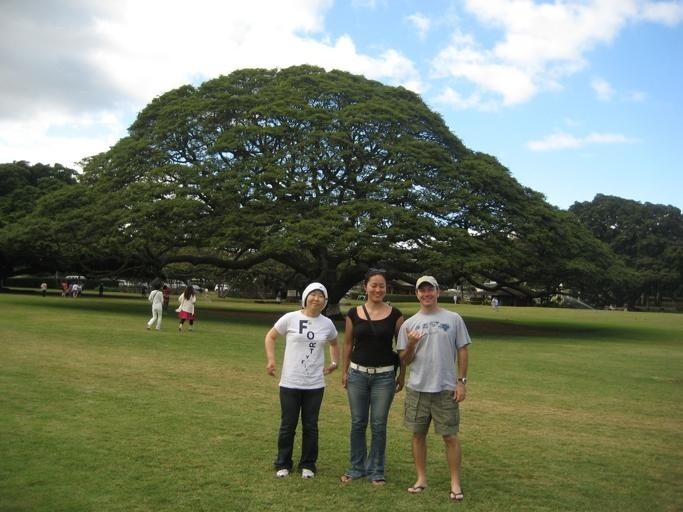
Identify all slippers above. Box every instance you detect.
[408,486,424,494]
[449,488,464,501]
[340,475,352,483]
[372,480,386,487]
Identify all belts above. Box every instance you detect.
[349,361,394,374]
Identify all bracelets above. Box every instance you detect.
[329,360,337,367]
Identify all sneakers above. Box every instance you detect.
[301,468,315,479]
[276,469,290,478]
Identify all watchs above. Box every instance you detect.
[456,378,467,384]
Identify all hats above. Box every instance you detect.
[302,282,329,312]
[416,276,439,290]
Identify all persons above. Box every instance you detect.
[39,281,47,295]
[141,285,147,298]
[146,281,164,332]
[454,294,457,304]
[274,287,283,304]
[161,285,171,312]
[265,280,339,482]
[174,285,196,333]
[203,286,209,299]
[197,285,202,298]
[491,295,501,311]
[98,282,104,297]
[394,272,473,500]
[338,272,404,487]
[58,280,84,298]
